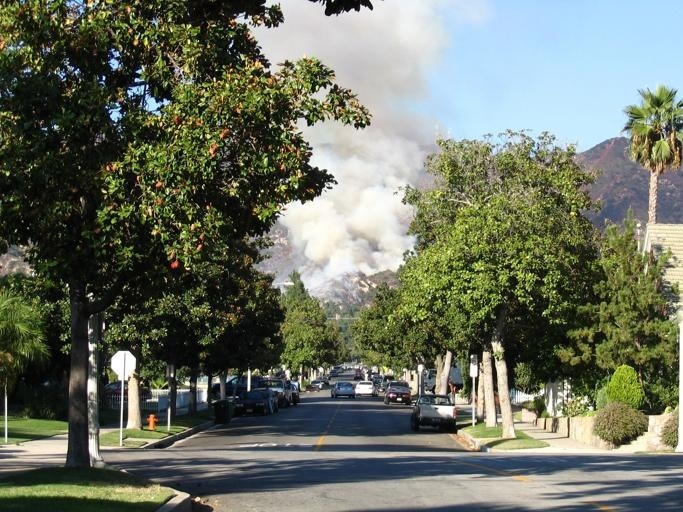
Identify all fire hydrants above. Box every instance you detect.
[146,413,158,429]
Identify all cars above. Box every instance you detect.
[306,364,344,391]
[353,363,411,405]
[105,381,153,403]
[210,375,313,391]
[330,380,357,399]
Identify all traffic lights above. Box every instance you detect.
[167,258,180,272]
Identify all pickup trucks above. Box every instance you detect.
[409,393,457,432]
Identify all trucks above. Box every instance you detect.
[424,368,461,394]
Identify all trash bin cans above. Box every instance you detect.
[209,399,231,424]
